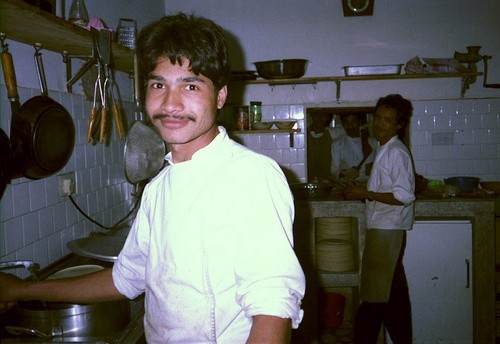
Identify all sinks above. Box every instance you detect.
[0,257,145,344]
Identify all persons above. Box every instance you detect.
[0,12,306,344]
[343,94,415,344]
[307,112,378,184]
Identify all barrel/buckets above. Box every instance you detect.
[319,294,346,331]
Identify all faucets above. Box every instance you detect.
[0,260,34,271]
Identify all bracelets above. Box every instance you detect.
[367,191,373,201]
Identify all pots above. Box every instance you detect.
[0,265,131,344]
[0,34,76,200]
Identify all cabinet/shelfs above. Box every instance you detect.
[227,71,484,134]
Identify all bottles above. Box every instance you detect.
[234,106,249,130]
[69,0,89,27]
[250,101,262,130]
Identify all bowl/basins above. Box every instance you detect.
[252,122,273,130]
[479,181,500,192]
[253,59,308,79]
[273,120,296,129]
[443,176,480,194]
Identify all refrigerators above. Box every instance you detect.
[385,219,472,344]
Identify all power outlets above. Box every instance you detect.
[58,171,76,197]
